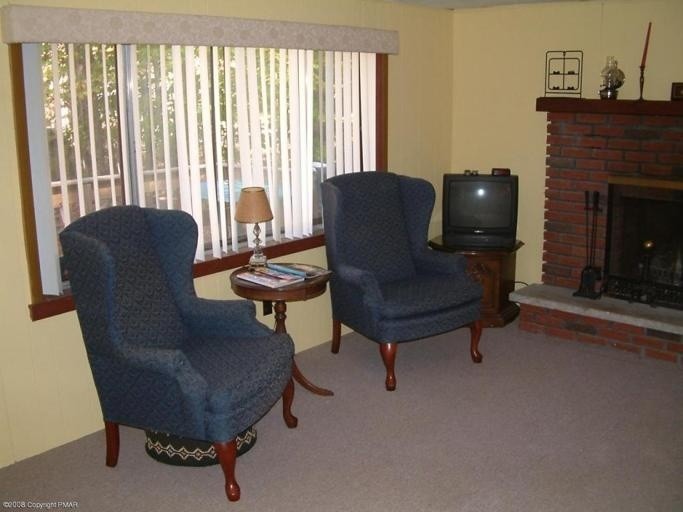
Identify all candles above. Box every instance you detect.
[637,21,652,99]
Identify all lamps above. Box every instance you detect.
[235,187,272,264]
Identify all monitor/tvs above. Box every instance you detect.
[442,173,518,249]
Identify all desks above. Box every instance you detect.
[428,235,523,328]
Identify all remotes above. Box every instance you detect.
[464,170,470,175]
[472,170,477,176]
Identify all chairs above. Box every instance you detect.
[321,172,484,390]
[60,206,297,501]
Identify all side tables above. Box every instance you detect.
[231,264,333,396]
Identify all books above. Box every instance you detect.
[236,263,332,289]
